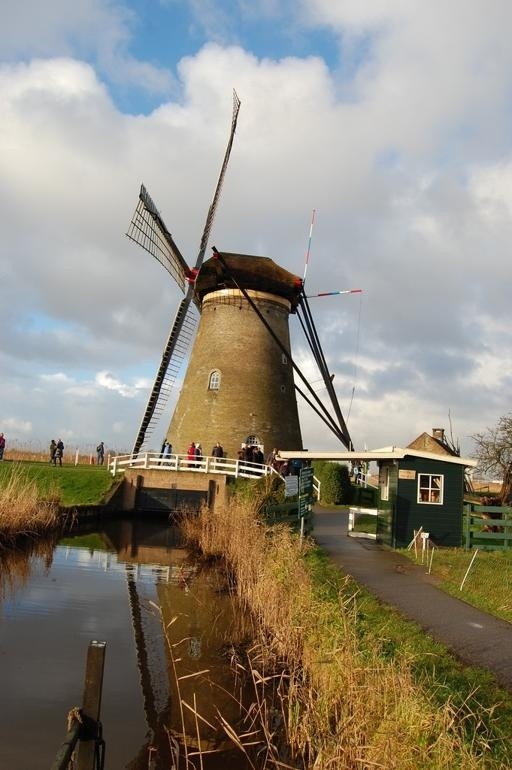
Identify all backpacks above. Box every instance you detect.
[96,445,103,453]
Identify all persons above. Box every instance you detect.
[157,437,302,478]
[96,442,104,465]
[50,438,64,467]
[0,432,5,461]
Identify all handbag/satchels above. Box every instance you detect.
[56,451,61,455]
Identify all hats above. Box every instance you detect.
[195,443,200,450]
